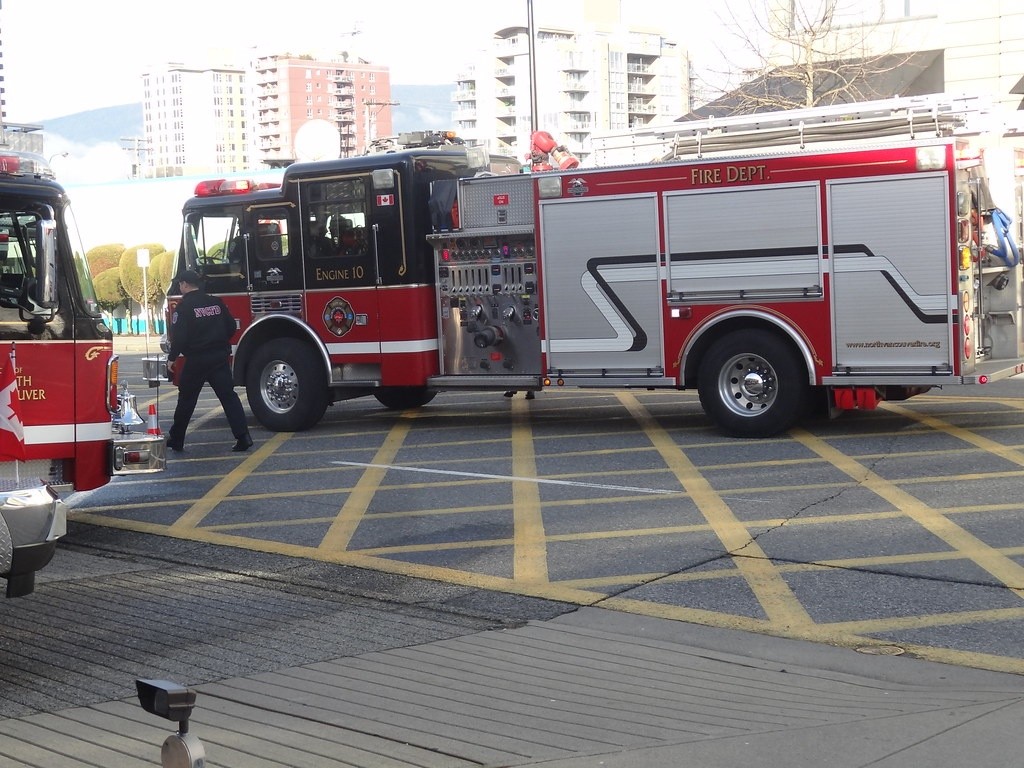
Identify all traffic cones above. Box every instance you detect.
[147,403,163,436]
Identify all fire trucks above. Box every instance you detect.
[0,142,139,601]
[140,88,1024,439]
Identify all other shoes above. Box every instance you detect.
[166,435,183,451]
[231,435,253,451]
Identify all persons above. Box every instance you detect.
[165,269,253,452]
[328,215,364,256]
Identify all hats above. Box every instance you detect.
[171,269,202,286]
